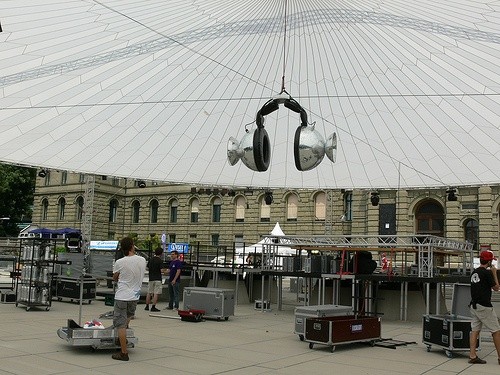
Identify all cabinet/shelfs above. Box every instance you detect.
[16,237,56,307]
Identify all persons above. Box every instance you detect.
[112,236,146,361]
[380,253,387,271]
[166,250,182,311]
[469,250,500,364]
[144,248,165,312]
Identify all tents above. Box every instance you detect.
[28,227,80,235]
[235,222,308,270]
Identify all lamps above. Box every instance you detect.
[446,187,457,201]
[206,189,211,195]
[198,189,204,194]
[213,189,219,195]
[191,188,196,194]
[371,192,379,206]
[221,189,228,196]
[38,170,46,177]
[139,181,146,188]
[264,191,273,205]
[228,189,235,196]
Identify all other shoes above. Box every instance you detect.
[112,353,129,361]
[174,305,179,308]
[167,307,172,310]
[151,308,160,311]
[469,356,486,364]
[145,307,149,310]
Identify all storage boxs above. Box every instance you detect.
[49,276,96,304]
[422,283,481,357]
[294,304,354,341]
[303,315,382,352]
[182,287,234,320]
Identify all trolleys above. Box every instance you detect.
[92,281,135,350]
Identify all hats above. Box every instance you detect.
[480,251,497,261]
